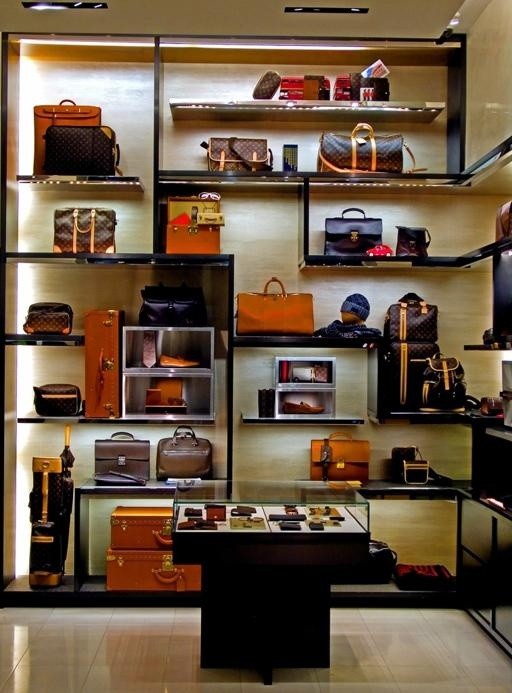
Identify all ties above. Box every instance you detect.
[143,330,157,368]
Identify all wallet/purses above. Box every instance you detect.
[314,367,328,383]
[178,504,325,531]
[329,481,347,489]
[93,471,146,485]
[346,480,362,488]
[257,389,274,418]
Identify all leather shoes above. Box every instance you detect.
[283,402,325,414]
[160,353,200,368]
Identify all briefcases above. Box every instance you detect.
[108,504,173,550]
[85,307,124,419]
[165,196,221,255]
[106,549,202,592]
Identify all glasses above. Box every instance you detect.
[198,191,222,201]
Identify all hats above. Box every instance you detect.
[341,294,370,321]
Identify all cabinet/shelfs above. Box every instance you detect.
[1,32,462,607]
[456,140,512,652]
[170,475,369,671]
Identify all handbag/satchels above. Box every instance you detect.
[53,207,116,254]
[95,432,150,480]
[156,379,182,405]
[316,123,428,174]
[138,285,209,327]
[369,540,398,580]
[324,207,382,257]
[22,302,73,335]
[391,446,414,482]
[29,456,65,588]
[403,460,430,485]
[196,212,225,226]
[201,138,273,172]
[33,98,122,176]
[253,70,390,101]
[157,426,212,481]
[310,432,370,483]
[237,277,315,336]
[395,225,431,257]
[33,385,81,416]
[380,292,469,413]
